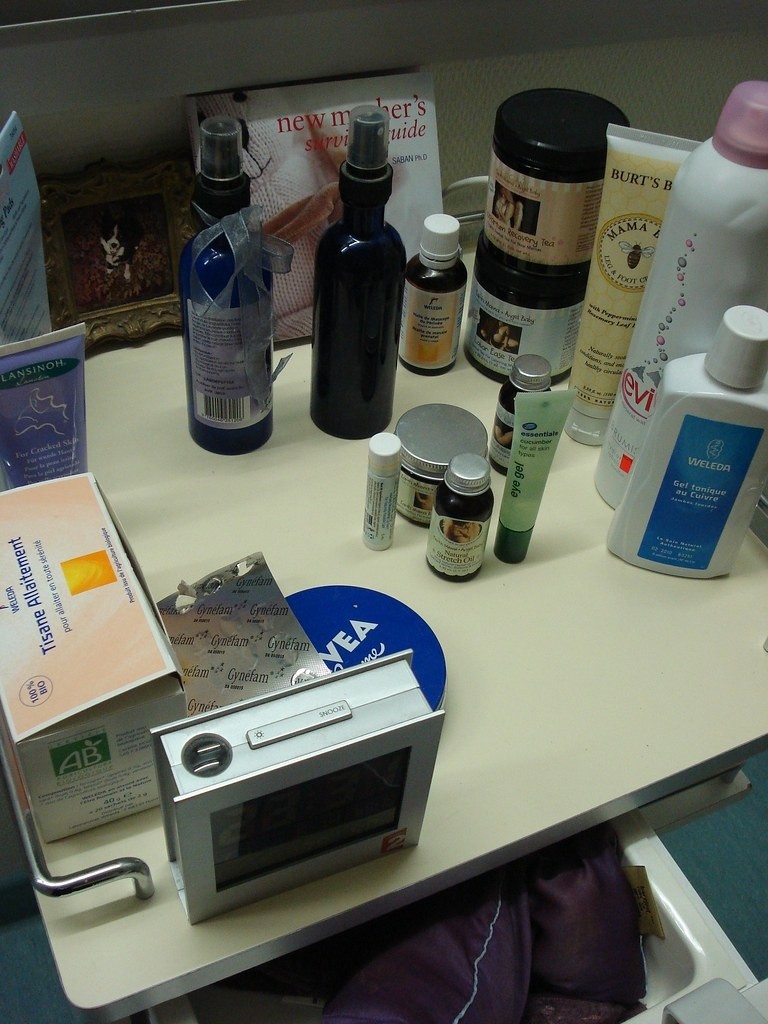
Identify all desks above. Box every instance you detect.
[0,252,768,1024]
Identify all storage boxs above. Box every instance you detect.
[0,472,187,844]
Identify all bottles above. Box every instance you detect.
[399,213,468,375]
[423,452,496,583]
[178,116,273,455]
[313,108,407,440]
[606,305,768,579]
[489,355,552,475]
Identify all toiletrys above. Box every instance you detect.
[0,322,87,489]
[310,102,408,440]
[177,114,272,458]
[560,120,704,448]
[604,306,768,583]
[493,390,576,565]
[594,78,768,511]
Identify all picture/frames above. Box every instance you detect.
[27,146,203,357]
